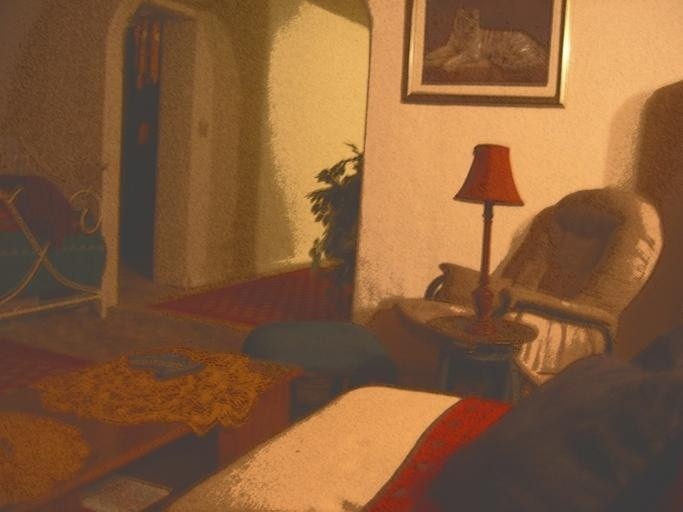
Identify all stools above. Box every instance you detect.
[237,319,404,422]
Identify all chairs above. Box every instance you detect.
[142,326,683,512]
[363,182,667,409]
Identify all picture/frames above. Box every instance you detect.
[400,1,570,112]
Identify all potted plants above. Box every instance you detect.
[305,139,363,317]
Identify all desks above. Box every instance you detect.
[423,317,537,411]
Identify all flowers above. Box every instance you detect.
[0,352,305,512]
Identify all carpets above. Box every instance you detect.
[147,259,355,325]
[0,337,87,393]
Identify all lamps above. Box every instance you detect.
[450,142,526,336]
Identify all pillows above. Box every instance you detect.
[427,345,681,512]
